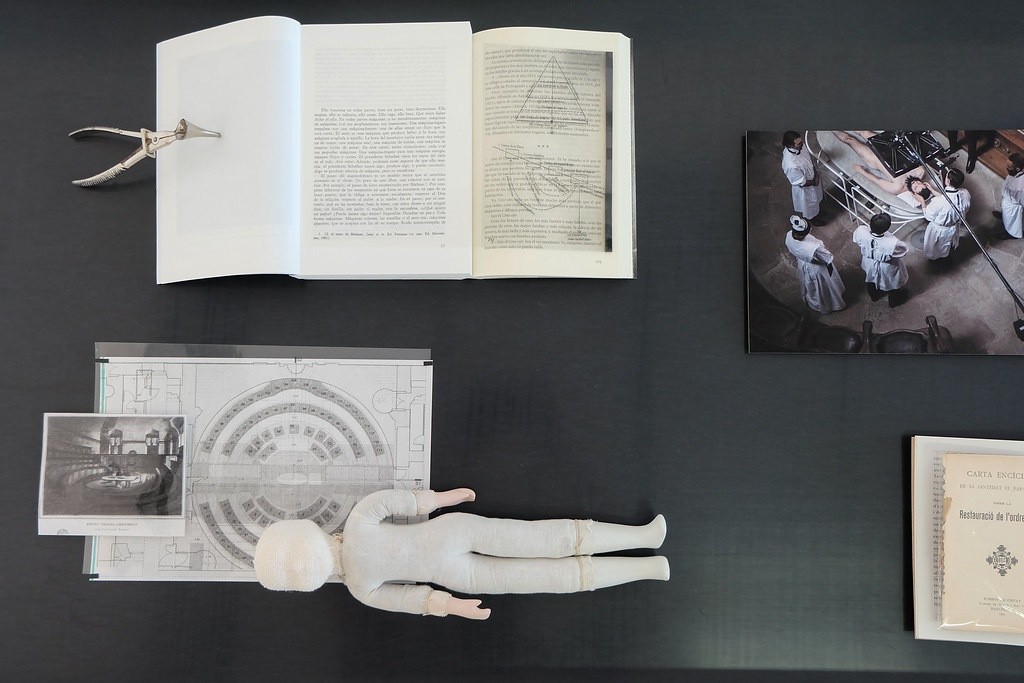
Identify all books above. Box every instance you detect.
[155,15,641,286]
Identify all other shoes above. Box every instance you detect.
[992,211,1002,219]
[871,290,887,301]
[811,219,824,226]
[996,232,1018,240]
[901,288,908,296]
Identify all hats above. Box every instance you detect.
[789,215,808,231]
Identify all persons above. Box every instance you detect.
[992,153,1024,239]
[253,489,671,621]
[781,131,978,314]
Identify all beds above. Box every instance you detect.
[804,130,949,235]
